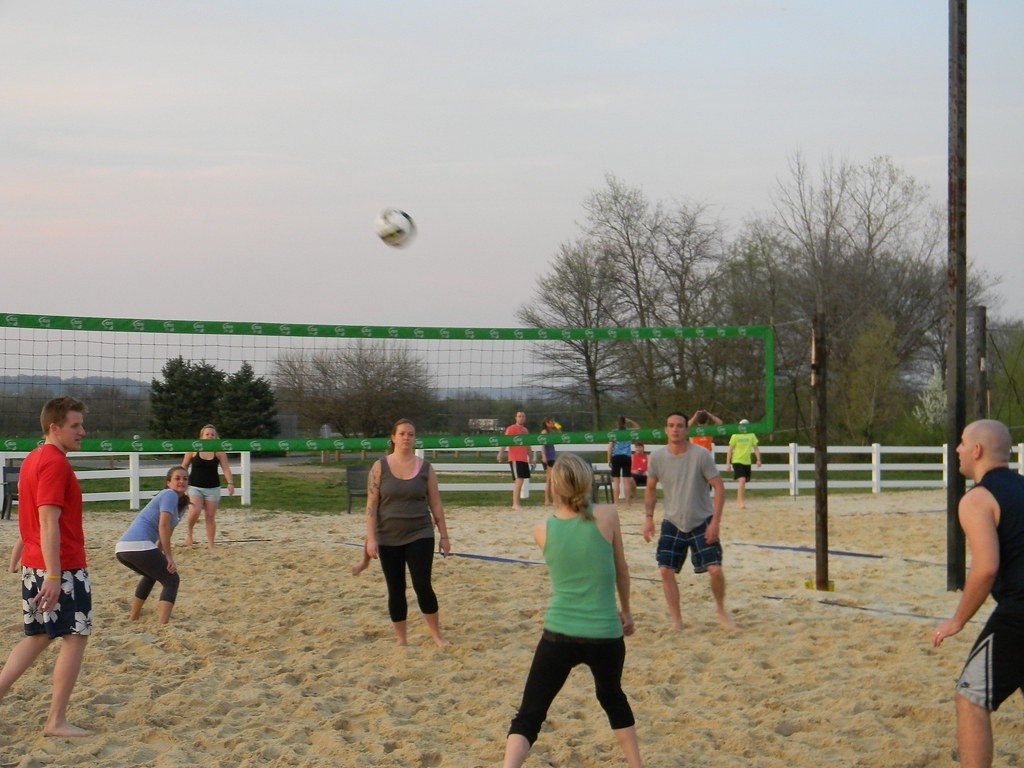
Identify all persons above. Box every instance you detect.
[497,409,537,510]
[181,425,235,547]
[727,420,761,509]
[0,395,94,737]
[541,418,556,506]
[607,416,647,508]
[503,452,640,768]
[352,418,451,648]
[687,409,723,451]
[932,419,1024,767]
[642,412,734,629]
[115,466,190,624]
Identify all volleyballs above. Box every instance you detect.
[374,206,419,250]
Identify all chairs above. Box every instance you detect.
[586,459,616,505]
[2,468,21,520]
[346,467,370,514]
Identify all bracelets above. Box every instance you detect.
[441,537,448,539]
[227,483,234,484]
[46,576,61,579]
[647,515,652,517]
